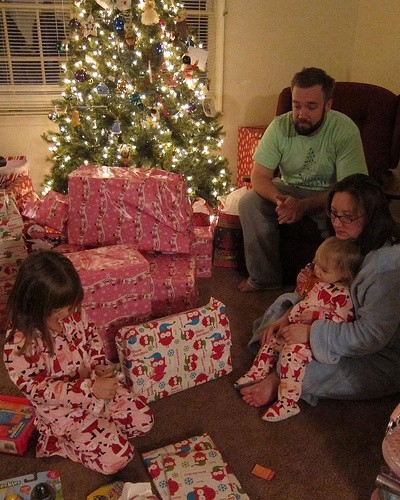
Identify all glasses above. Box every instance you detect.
[326,209,363,224]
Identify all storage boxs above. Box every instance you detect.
[63,245,153,360]
[212,221,242,273]
[237,125,266,191]
[192,199,211,227]
[193,225,214,278]
[141,433,250,500]
[0,155,68,332]
[0,394,36,456]
[141,252,198,320]
[215,201,241,229]
[115,296,233,404]
[68,165,194,254]
[63,245,153,359]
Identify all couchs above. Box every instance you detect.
[276,81,400,287]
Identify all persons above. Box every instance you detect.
[3,250,154,476]
[233,238,355,423]
[237,68,370,294]
[240,173,400,407]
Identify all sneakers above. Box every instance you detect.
[261,399,300,422]
[234,371,267,390]
